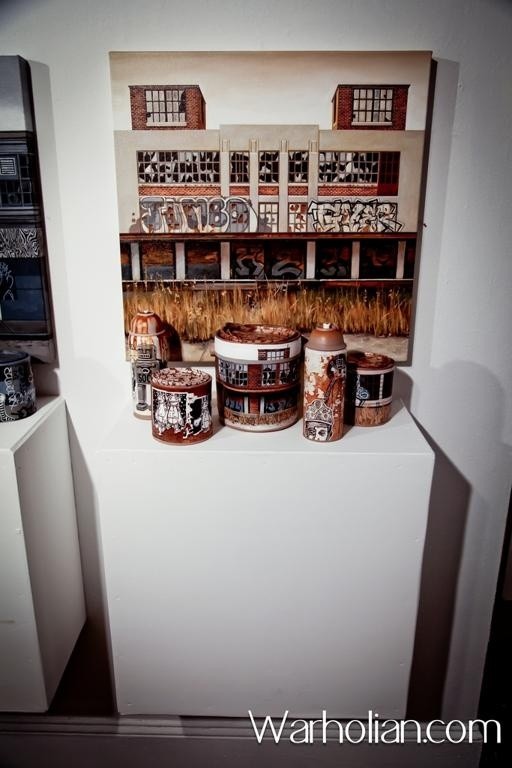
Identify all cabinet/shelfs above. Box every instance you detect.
[2,398,85,712]
[101,400,435,721]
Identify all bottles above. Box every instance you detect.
[302,321,347,443]
[149,368,215,445]
[211,325,302,434]
[348,352,396,428]
[126,310,170,422]
[1,346,36,425]
